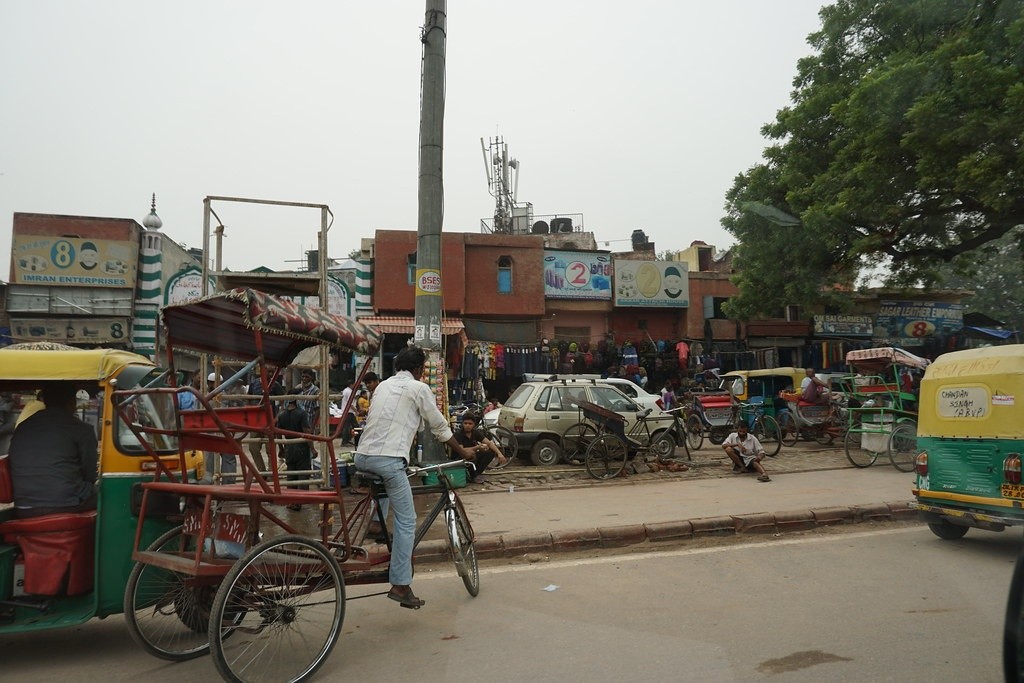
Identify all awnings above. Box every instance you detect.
[960,324,1019,340]
[359,316,464,336]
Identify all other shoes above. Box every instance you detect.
[733,469,745,474]
[341,443,353,447]
[287,504,301,511]
[757,474,769,481]
[253,475,267,482]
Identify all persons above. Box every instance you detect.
[0,365,506,512]
[354,346,481,606]
[661,380,678,411]
[722,420,770,481]
[802,367,840,404]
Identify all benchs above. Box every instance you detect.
[179,406,338,501]
[852,383,917,414]
[794,395,827,408]
[692,392,733,408]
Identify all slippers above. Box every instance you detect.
[468,477,484,484]
[366,529,393,540]
[388,588,425,605]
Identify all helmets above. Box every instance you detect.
[208,372,223,381]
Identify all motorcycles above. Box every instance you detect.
[907,343,1024,540]
[0,345,239,633]
[708,367,809,445]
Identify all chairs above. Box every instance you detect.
[561,391,588,410]
[0,454,98,597]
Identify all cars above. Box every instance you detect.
[479,378,667,442]
[815,374,854,401]
[495,374,685,467]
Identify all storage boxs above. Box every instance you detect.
[312,457,348,488]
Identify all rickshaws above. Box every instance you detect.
[123,287,481,683]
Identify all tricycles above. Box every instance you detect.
[559,399,692,480]
[685,387,782,457]
[352,402,518,469]
[777,386,862,448]
[838,347,931,473]
